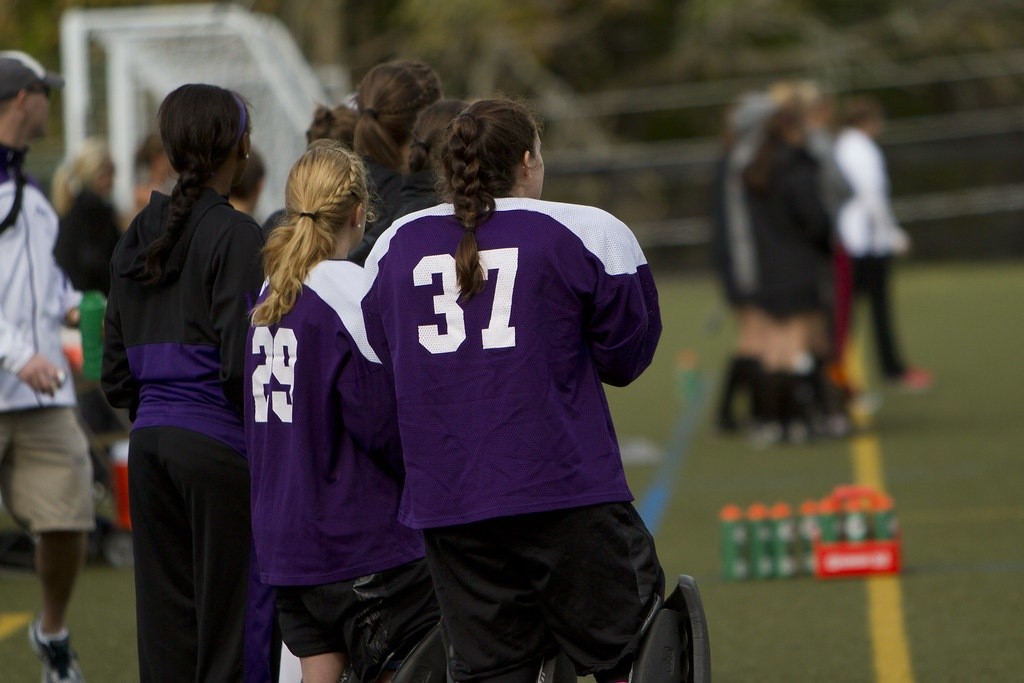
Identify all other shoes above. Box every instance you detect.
[717,350,856,450]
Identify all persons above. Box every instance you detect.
[0,48,470,683]
[358,99,665,683]
[713,76,932,447]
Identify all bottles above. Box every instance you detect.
[722,500,897,580]
[79,291,106,379]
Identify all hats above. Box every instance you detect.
[0,49,65,102]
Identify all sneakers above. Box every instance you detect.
[885,363,929,389]
[28,612,86,683]
